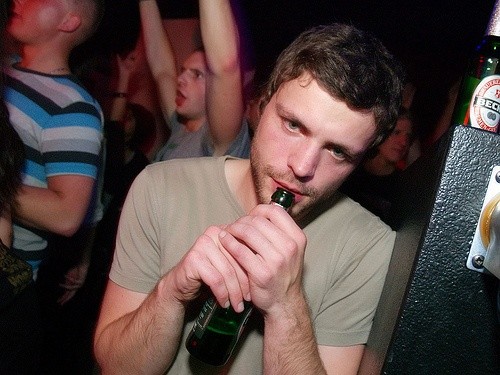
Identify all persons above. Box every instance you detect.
[95,24,398,375]
[0,0,491,375]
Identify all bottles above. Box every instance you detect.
[455,1,499,133]
[184,187,295,367]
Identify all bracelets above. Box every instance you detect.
[111,91,128,97]
